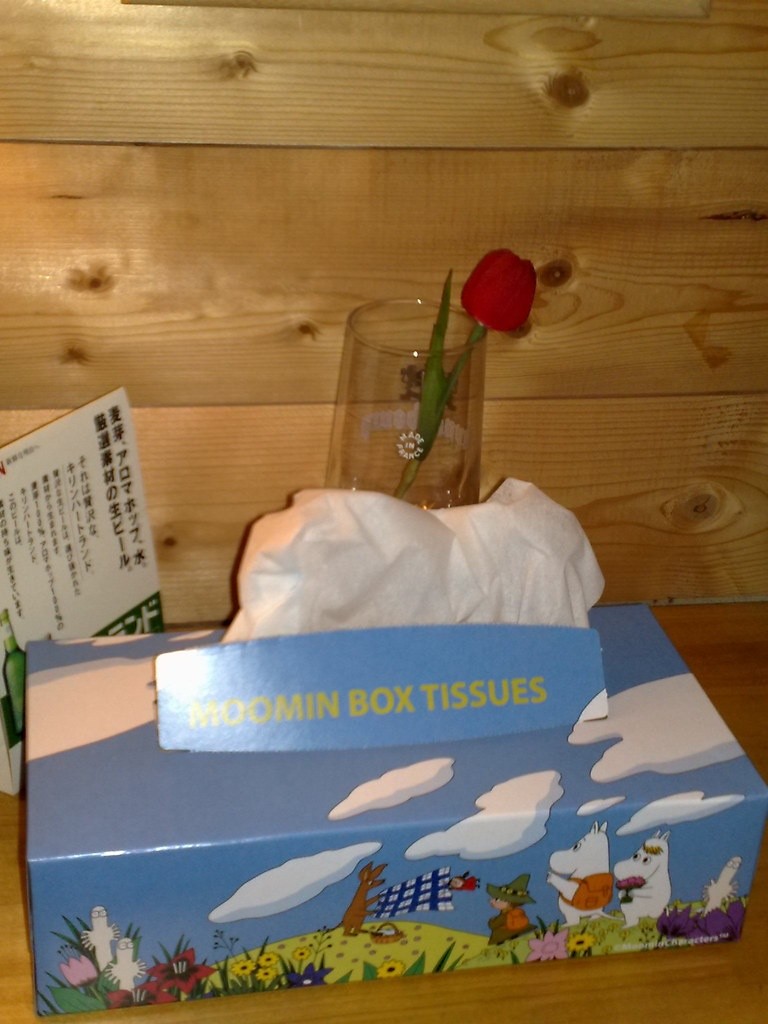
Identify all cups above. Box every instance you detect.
[327,298,489,512]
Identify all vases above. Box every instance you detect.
[323,296,488,510]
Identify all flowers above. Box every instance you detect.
[397,248,538,498]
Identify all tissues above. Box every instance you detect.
[24,476,767,1013]
